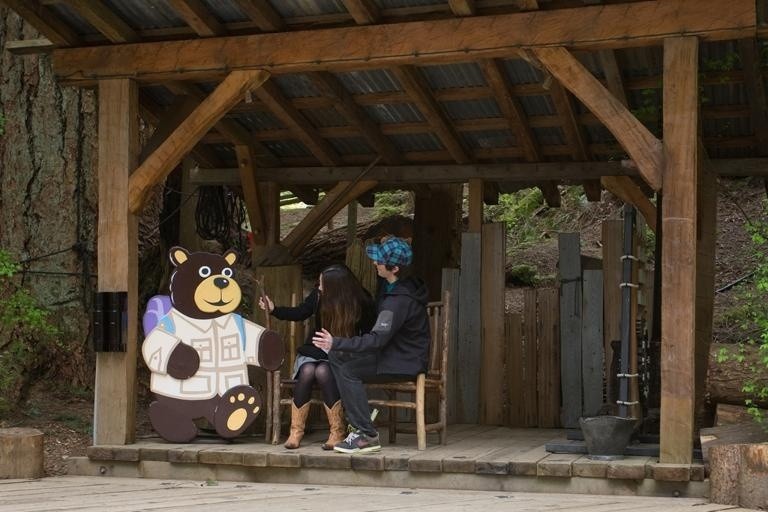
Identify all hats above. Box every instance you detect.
[366,237,414,267]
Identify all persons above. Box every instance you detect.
[258,263,378,451]
[310,235,434,452]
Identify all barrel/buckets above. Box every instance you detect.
[577,415,637,459]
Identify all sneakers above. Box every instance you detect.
[332,431,382,454]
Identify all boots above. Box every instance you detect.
[321,399,347,450]
[283,397,312,449]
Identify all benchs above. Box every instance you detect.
[263,290,451,448]
